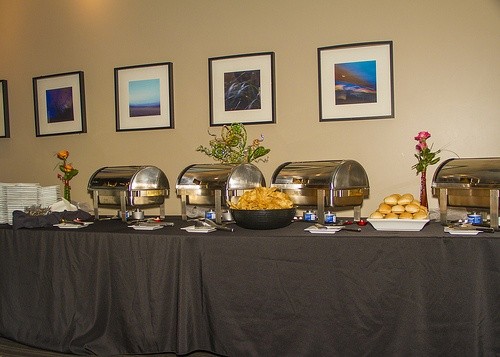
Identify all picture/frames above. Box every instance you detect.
[0,79,11,138]
[32,71,86,137]
[114,62,174,132]
[317,40,394,122]
[208,52,275,127]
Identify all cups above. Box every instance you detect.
[303,211,318,221]
[132,210,144,219]
[204,210,216,220]
[222,211,233,221]
[467,214,483,225]
[324,211,337,223]
[117,210,131,219]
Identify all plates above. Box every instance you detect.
[0,182,61,227]
[443,226,484,235]
[52,222,95,229]
[367,212,431,232]
[127,225,164,231]
[180,225,217,233]
[304,225,346,233]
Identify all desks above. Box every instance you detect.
[0,216,500,357]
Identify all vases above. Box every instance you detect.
[420,168,428,210]
[64,180,71,201]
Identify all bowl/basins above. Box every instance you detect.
[229,208,296,230]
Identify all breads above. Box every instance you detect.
[368,193,429,219]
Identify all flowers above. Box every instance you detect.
[410,130,460,176]
[52,150,78,182]
